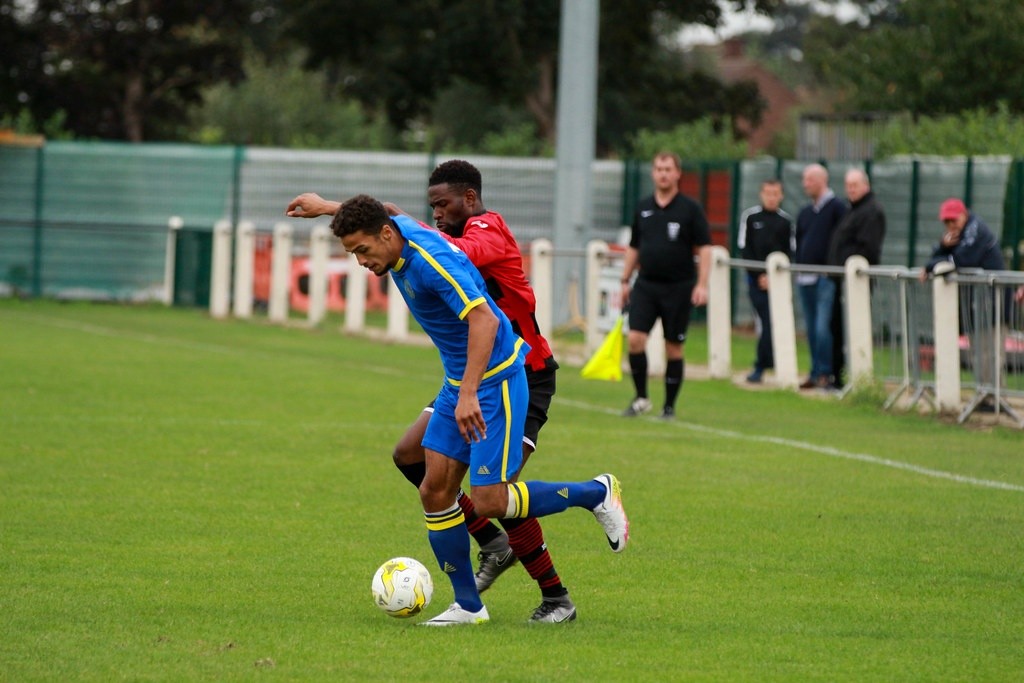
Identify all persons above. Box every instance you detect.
[383,160,577,624]
[796,164,848,390]
[286,191,630,627]
[737,180,792,385]
[827,167,887,391]
[921,196,1016,413]
[616,154,713,420]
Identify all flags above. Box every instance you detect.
[581,320,623,382]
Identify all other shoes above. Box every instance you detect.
[801,372,848,393]
[745,365,766,382]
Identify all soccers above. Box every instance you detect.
[371,556,434,619]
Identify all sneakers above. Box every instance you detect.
[618,396,653,418]
[474,545,521,595]
[590,473,629,553]
[415,602,490,628]
[525,594,580,627]
[650,406,677,424]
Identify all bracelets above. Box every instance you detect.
[620,279,629,283]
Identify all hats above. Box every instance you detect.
[938,198,968,222]
